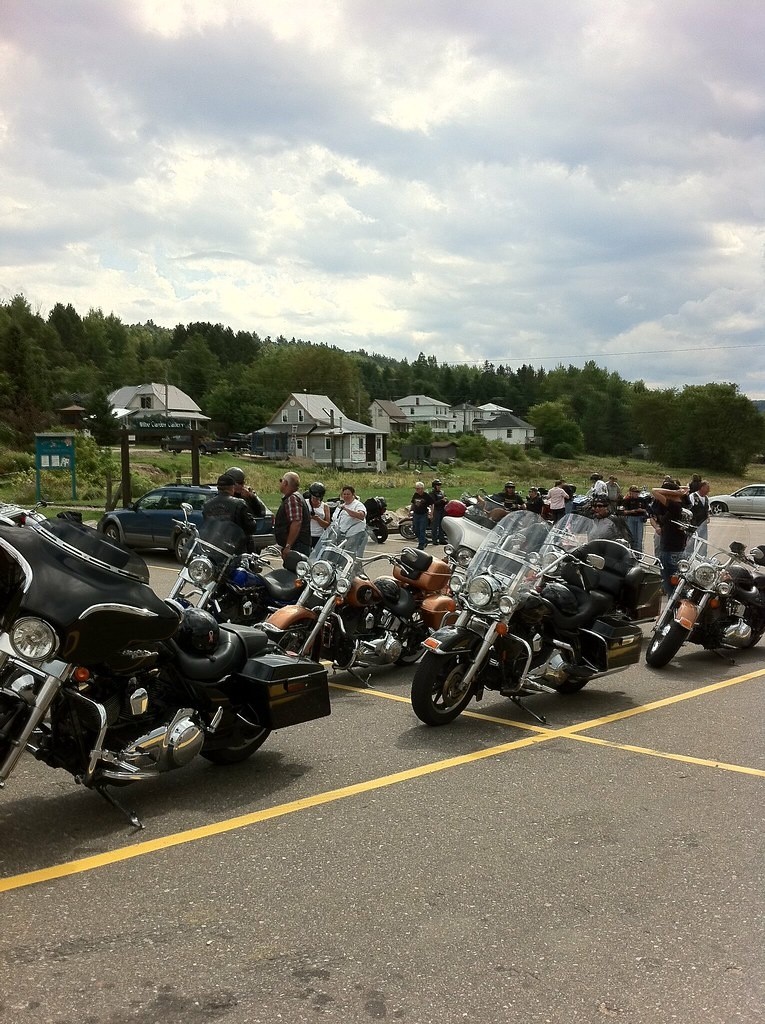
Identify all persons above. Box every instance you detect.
[543,472,647,553]
[411,482,435,551]
[331,486,367,569]
[203,466,267,555]
[274,472,311,571]
[304,481,330,549]
[526,487,545,517]
[488,481,527,512]
[428,479,449,545]
[650,472,711,599]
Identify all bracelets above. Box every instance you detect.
[285,544,290,549]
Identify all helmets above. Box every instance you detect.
[309,482,326,498]
[432,479,442,487]
[505,481,515,487]
[591,473,599,480]
[224,466,246,481]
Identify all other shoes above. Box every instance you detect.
[432,540,439,545]
[416,538,430,550]
[438,540,448,545]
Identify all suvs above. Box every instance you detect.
[99,483,277,563]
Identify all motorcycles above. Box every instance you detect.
[398,489,664,729]
[0,497,332,831]
[327,497,392,542]
[646,507,765,667]
[165,501,451,690]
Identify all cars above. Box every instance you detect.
[160,431,250,454]
[708,484,765,519]
[638,444,648,449]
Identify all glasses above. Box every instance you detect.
[415,488,421,490]
[279,478,285,482]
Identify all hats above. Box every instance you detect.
[629,485,642,493]
[217,473,237,486]
[608,475,617,480]
